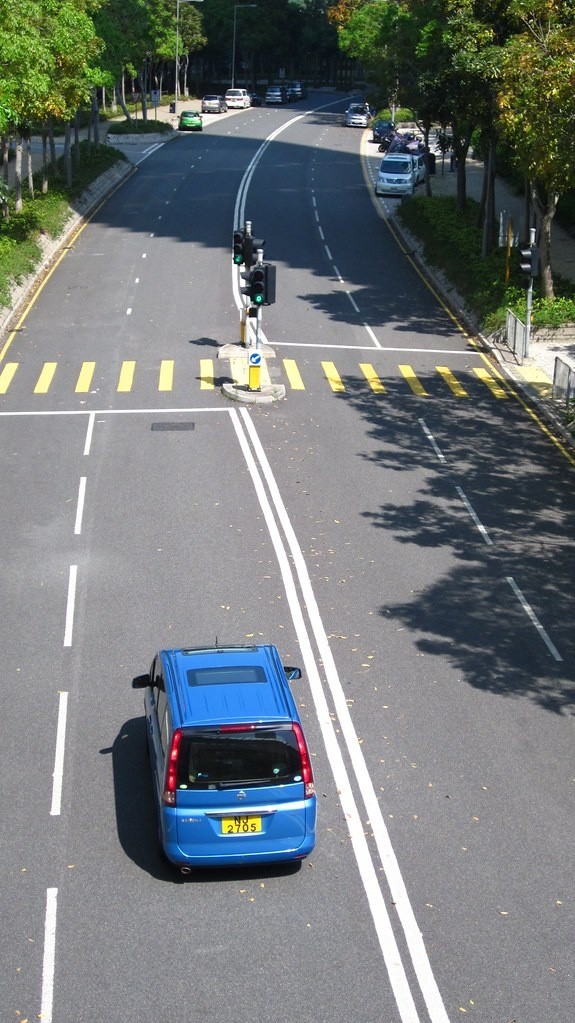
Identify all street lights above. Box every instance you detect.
[230,4,257,89]
[174,0,204,117]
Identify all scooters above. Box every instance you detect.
[378,129,430,156]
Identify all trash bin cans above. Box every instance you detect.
[170,103,175,113]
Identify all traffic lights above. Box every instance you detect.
[250,267,269,305]
[518,246,541,278]
[239,270,252,297]
[233,228,246,267]
[247,237,266,269]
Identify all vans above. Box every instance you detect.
[265,82,305,105]
[225,89,251,109]
[132,643,318,876]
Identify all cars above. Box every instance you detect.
[248,92,262,107]
[201,95,228,114]
[177,110,203,131]
[371,120,398,143]
[346,103,370,127]
[374,152,427,198]
